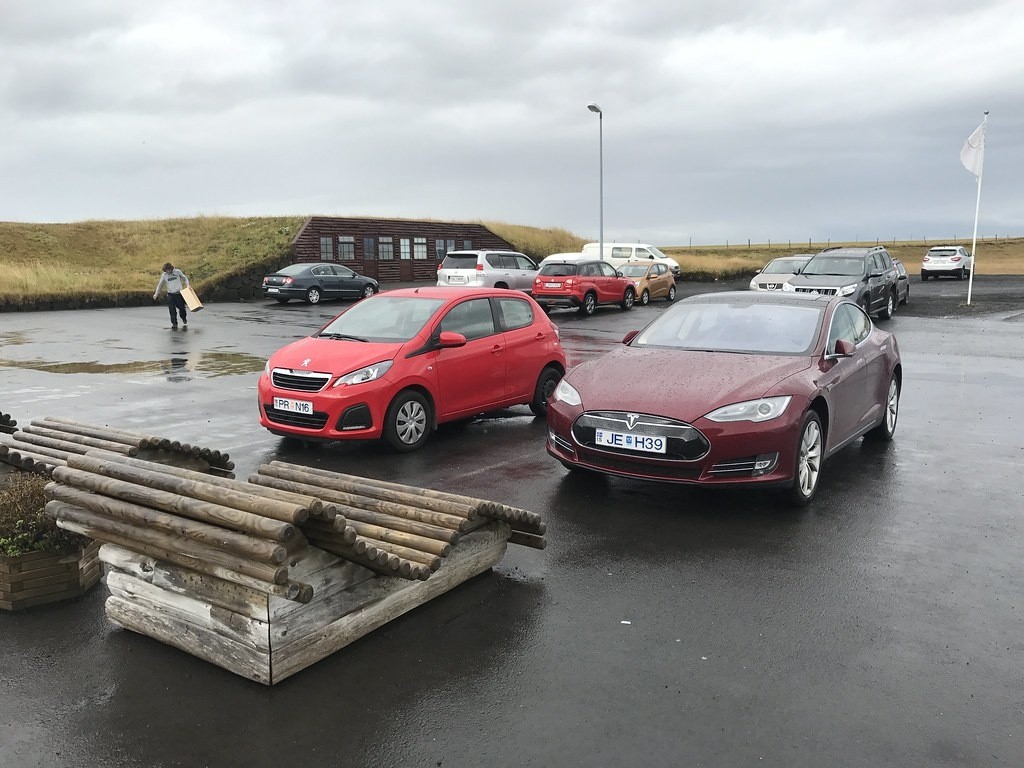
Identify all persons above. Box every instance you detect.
[153,263,189,328]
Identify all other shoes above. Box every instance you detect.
[183,322,187,327]
[172,322,178,328]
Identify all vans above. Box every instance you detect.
[582,243,680,282]
[436,248,540,299]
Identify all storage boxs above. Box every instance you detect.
[180,286,204,312]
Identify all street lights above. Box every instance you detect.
[587,102,602,258]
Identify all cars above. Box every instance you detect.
[538,252,581,268]
[616,261,676,304]
[749,256,812,292]
[546,291,902,507]
[262,263,379,304]
[257,286,566,453]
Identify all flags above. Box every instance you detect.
[960,120,984,182]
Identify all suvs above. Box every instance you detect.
[783,246,898,321]
[532,259,638,317]
[921,246,975,281]
